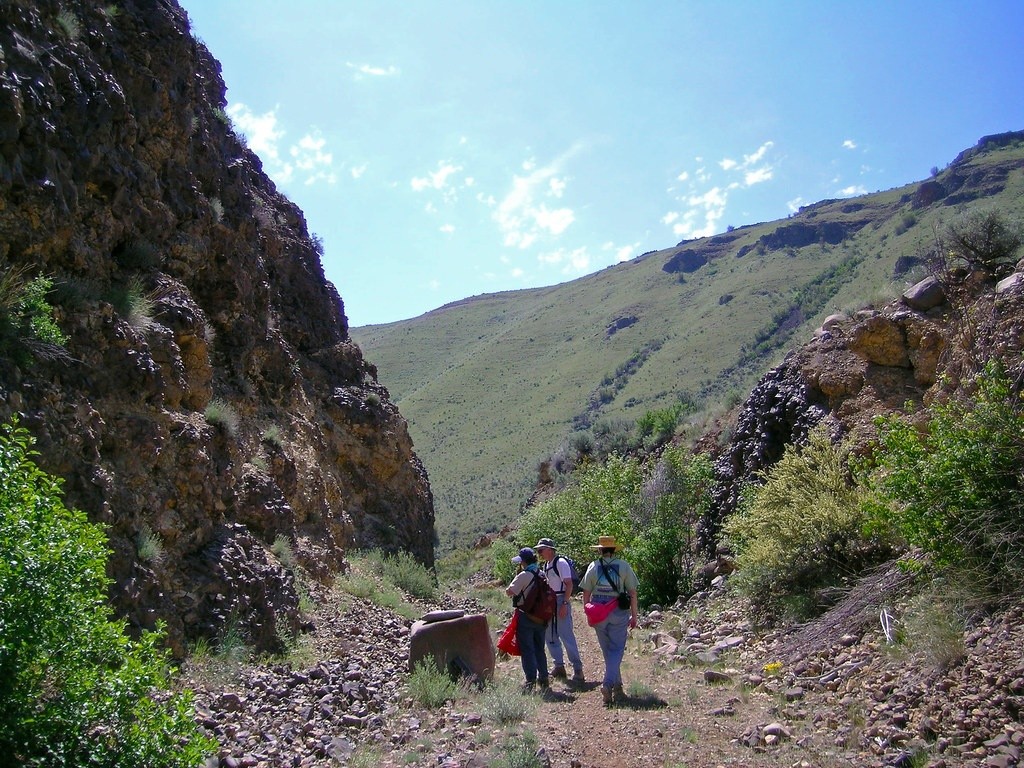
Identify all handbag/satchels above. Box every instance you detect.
[617,592,631,610]
[584,598,618,626]
[497,609,521,656]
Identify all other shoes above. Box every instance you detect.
[518,681,536,692]
[601,683,613,704]
[613,687,629,702]
[536,676,549,687]
[568,671,586,685]
[550,666,566,680]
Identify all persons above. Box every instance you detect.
[578,536,640,705]
[533,538,585,687]
[506,548,552,696]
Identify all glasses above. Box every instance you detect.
[538,549,544,553]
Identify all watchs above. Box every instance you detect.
[563,601,568,605]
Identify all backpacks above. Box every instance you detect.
[520,569,557,624]
[543,556,581,595]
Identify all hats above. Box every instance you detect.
[590,535,624,552]
[511,547,534,563]
[533,538,557,551]
